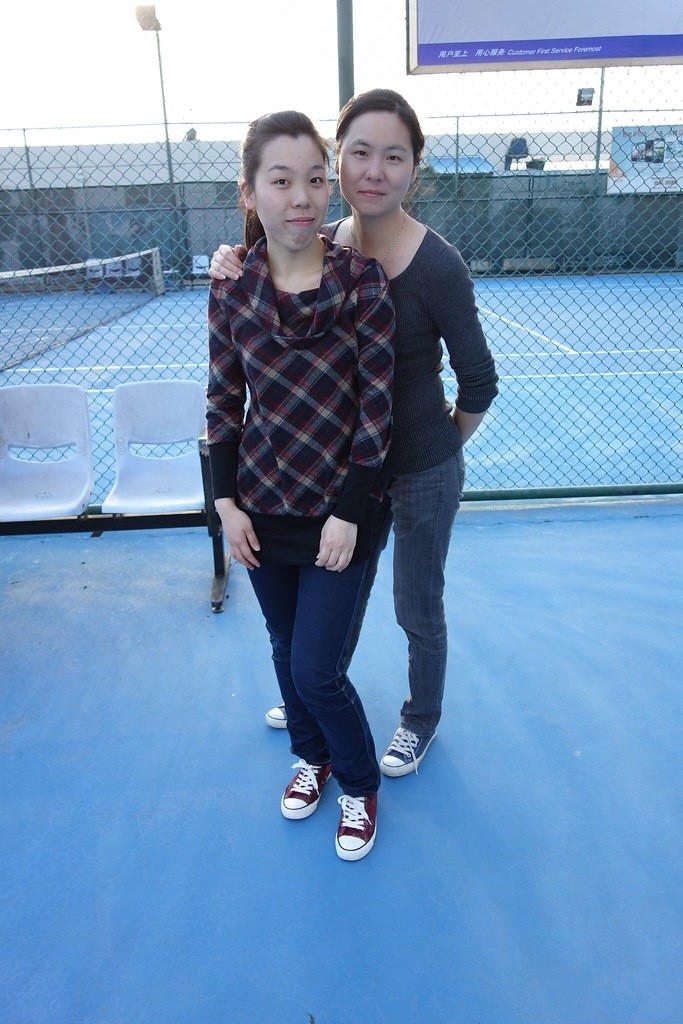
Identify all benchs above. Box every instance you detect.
[84,257,143,294]
[470,258,489,272]
[503,256,556,273]
[0,379,230,613]
[189,255,210,288]
[675,250,683,267]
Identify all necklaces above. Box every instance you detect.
[349,212,405,267]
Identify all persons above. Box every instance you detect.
[208,88,500,778]
[204,110,396,862]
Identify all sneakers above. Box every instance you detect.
[335,787,377,861]
[280,759,334,819]
[265,703,288,728]
[379,728,437,777]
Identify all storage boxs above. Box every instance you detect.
[162,270,180,287]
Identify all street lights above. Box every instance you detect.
[135,4,189,281]
